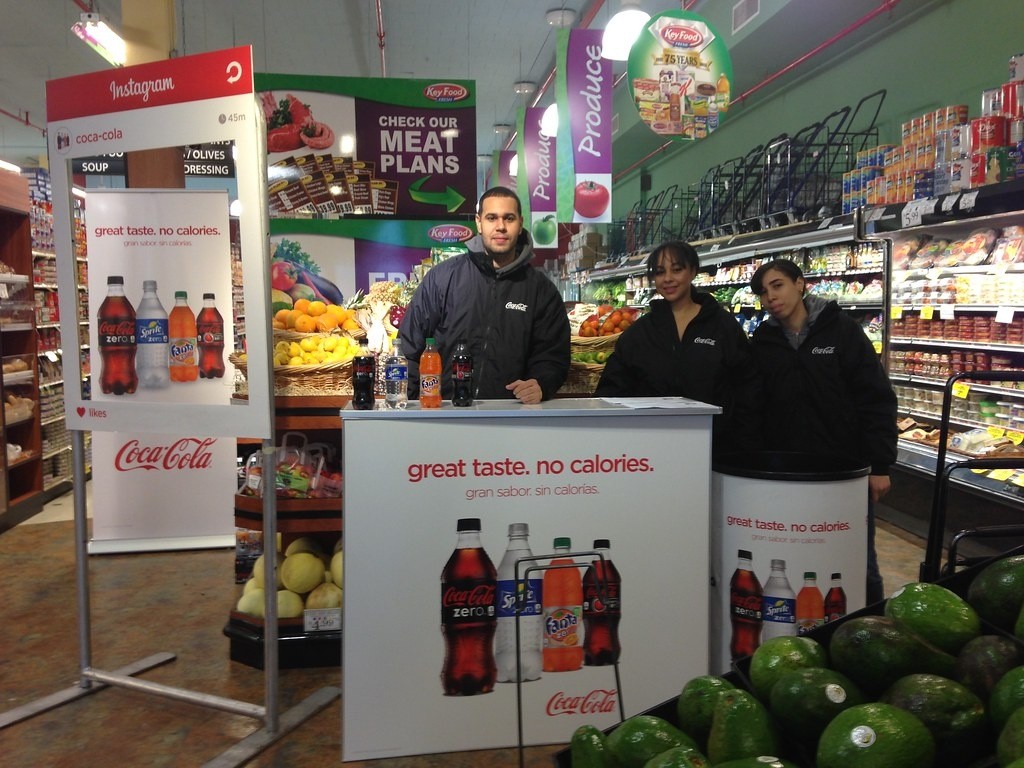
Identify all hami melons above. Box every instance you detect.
[238,531,346,617]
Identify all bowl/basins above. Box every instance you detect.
[891,385,1024,432]
[989,355,1024,390]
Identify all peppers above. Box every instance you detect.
[270,261,323,306]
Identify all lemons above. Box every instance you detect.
[572,352,613,366]
[238,336,365,368]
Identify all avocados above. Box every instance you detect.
[572,556,1023,768]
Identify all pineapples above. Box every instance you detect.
[364,280,402,307]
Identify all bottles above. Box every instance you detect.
[195,293,225,378]
[670,93,681,121]
[542,537,584,672]
[135,280,172,387]
[716,73,730,111]
[168,291,199,381]
[492,523,542,681]
[729,550,848,661]
[419,338,442,408]
[708,103,719,136]
[451,337,473,408]
[352,339,375,410]
[384,339,409,408]
[845,242,873,268]
[440,518,499,695]
[581,539,621,665]
[97,276,139,394]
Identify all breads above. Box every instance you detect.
[1,261,34,423]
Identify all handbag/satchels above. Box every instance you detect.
[236,431,343,498]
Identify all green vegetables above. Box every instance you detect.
[270,236,322,278]
[592,278,626,309]
[266,98,317,137]
[708,286,739,303]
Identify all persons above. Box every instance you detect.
[750,260,899,603]
[396,186,571,404]
[595,240,760,472]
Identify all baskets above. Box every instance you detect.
[229,310,380,394]
[564,325,623,389]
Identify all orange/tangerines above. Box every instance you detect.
[578,306,632,336]
[270,299,360,330]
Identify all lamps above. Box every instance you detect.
[541,100,564,138]
[512,80,537,95]
[493,123,512,135]
[600,0,653,62]
[478,155,492,163]
[544,6,578,28]
[80,12,127,68]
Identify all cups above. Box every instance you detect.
[887,349,990,386]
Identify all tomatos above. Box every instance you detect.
[574,181,610,218]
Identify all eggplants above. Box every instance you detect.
[287,261,344,306]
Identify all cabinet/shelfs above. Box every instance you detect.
[0,166,1024,675]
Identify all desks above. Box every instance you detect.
[339,396,721,762]
